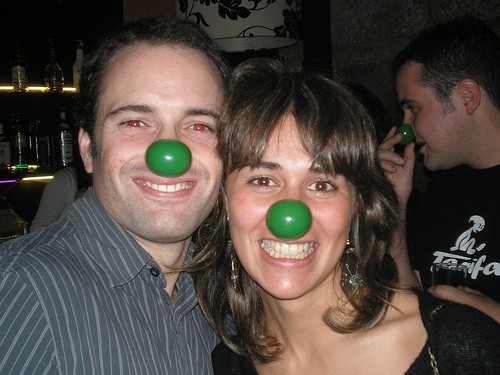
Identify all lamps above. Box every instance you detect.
[174,0,302,55]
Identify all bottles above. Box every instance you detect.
[12,54,25,84]
[44,36,66,86]
[0,106,76,172]
[72,39,85,86]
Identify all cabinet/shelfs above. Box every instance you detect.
[0,83,88,186]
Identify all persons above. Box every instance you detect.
[375,19,500,322]
[29,164,93,234]
[170,57,500,375]
[0,16,236,375]
